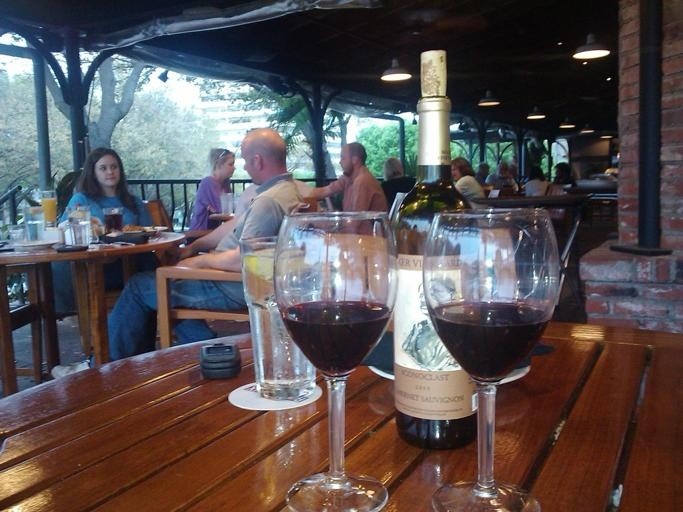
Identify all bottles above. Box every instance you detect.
[397,51,481,450]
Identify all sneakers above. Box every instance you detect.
[51,360,89,379]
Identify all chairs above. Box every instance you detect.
[156,198,319,350]
[147,199,215,239]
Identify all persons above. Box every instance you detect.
[59,148,216,345]
[310,144,389,235]
[382,157,416,211]
[524,165,551,197]
[554,163,577,187]
[51,129,307,382]
[475,162,490,185]
[451,157,488,210]
[510,155,518,179]
[190,148,234,233]
[482,163,518,192]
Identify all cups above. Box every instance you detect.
[42,190,58,226]
[220,193,234,217]
[7,226,25,246]
[68,210,91,244]
[273,210,398,508]
[23,207,45,242]
[423,208,560,508]
[103,207,124,234]
[240,237,324,403]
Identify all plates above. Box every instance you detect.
[102,232,155,243]
[143,226,169,231]
[367,364,530,385]
[9,240,60,251]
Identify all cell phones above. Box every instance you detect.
[198,339,244,381]
[56,243,89,252]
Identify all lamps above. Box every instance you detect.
[571,34,610,61]
[558,115,577,131]
[581,121,614,141]
[527,106,547,121]
[380,58,413,83]
[477,89,501,108]
